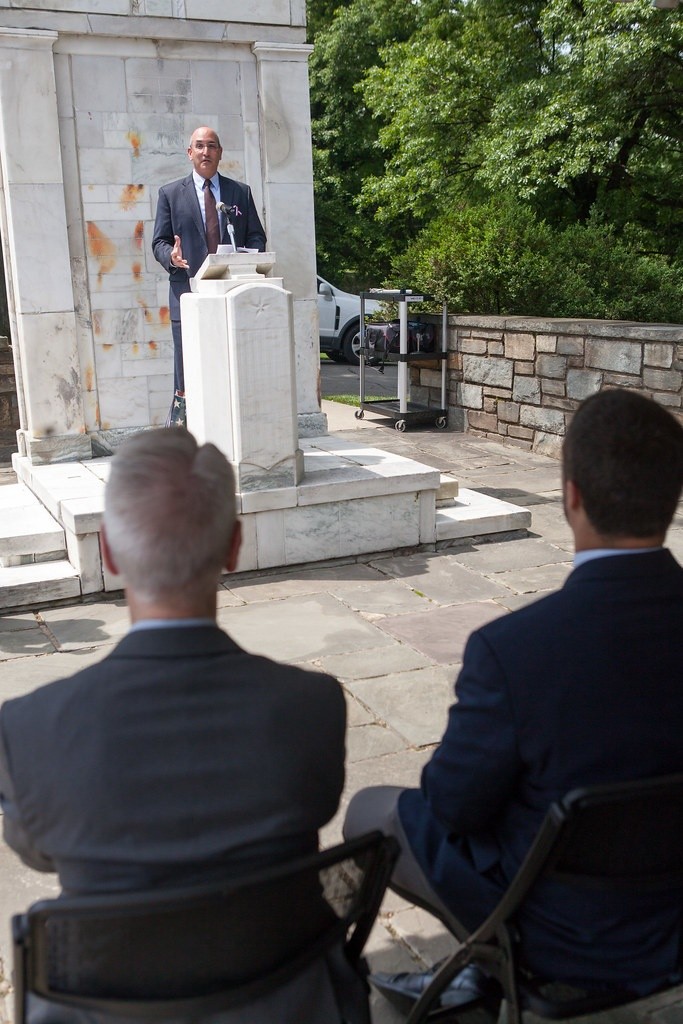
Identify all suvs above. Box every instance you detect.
[318,275,398,367]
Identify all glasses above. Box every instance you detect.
[191,143,219,152]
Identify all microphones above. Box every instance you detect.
[215,202,236,215]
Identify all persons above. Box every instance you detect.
[341,390,683,1007]
[151,127,267,427]
[0,429,373,1024]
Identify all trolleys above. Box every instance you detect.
[355,286,450,431]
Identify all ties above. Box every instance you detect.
[203,179,221,255]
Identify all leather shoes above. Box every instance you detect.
[369,956,504,1024]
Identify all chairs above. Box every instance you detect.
[15,830,403,1024]
[401,780,683,1024]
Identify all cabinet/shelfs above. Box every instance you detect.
[354,292,449,432]
